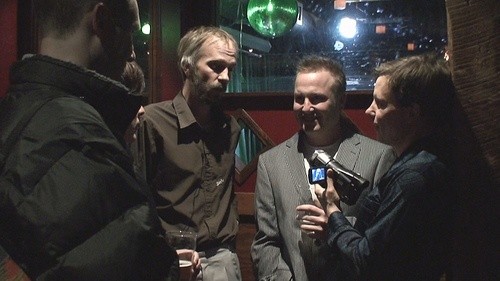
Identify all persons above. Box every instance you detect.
[1,0,242,281]
[312,168,325,182]
[251,53,496,281]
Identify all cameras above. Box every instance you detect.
[308,149,369,205]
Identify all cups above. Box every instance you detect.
[298,185,325,233]
[166,231,198,268]
